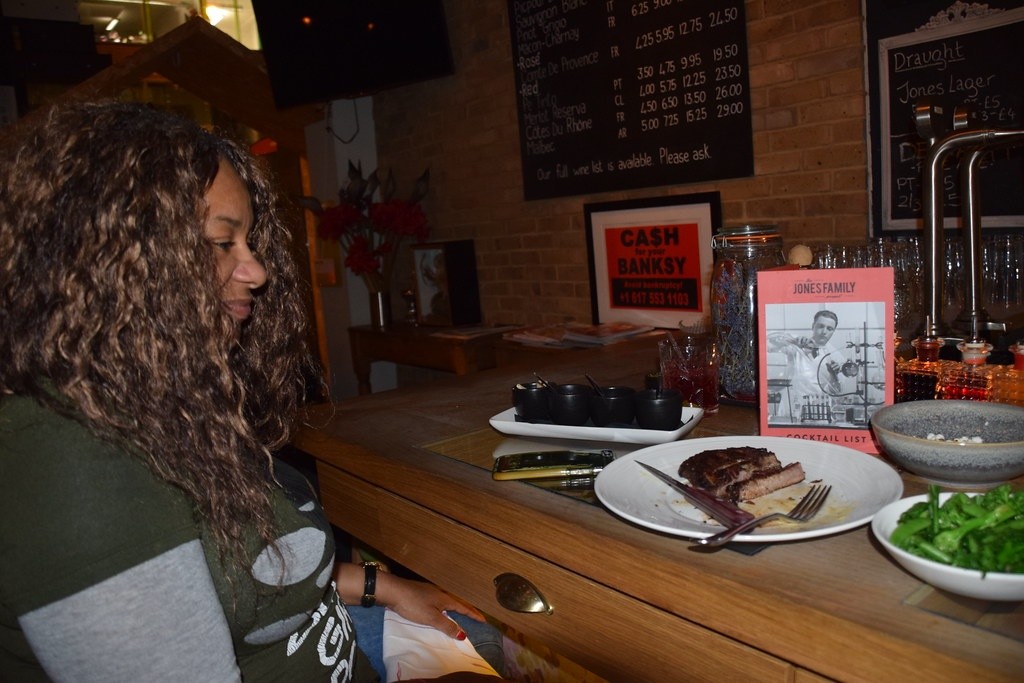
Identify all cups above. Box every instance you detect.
[656,337,720,417]
[513,380,685,429]
[813,232,1024,338]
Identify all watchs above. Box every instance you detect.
[359,561,383,607]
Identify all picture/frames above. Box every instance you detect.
[579,189,727,330]
[871,0,1024,234]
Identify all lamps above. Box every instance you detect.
[105,15,120,32]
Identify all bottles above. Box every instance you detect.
[710,226,787,406]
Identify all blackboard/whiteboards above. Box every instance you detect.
[878,4,1024,230]
[505,1,756,198]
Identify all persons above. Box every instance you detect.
[767,310,845,416]
[1,75,504,683]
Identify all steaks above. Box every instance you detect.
[677,446,806,504]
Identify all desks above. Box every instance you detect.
[487,330,684,369]
[345,320,521,398]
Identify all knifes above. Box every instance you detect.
[634,458,755,531]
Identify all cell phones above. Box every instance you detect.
[491,450,614,481]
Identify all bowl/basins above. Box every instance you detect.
[871,400,1024,485]
[872,492,1024,601]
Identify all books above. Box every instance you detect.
[502,322,655,347]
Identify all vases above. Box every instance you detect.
[365,291,395,331]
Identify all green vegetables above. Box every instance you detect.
[889,482,1024,579]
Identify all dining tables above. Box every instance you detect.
[279,318,1024,683]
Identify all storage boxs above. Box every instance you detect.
[407,241,485,328]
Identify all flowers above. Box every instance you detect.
[287,157,435,290]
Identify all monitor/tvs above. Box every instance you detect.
[252,0,455,109]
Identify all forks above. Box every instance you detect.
[689,485,833,549]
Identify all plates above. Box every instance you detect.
[486,406,703,445]
[593,434,904,543]
